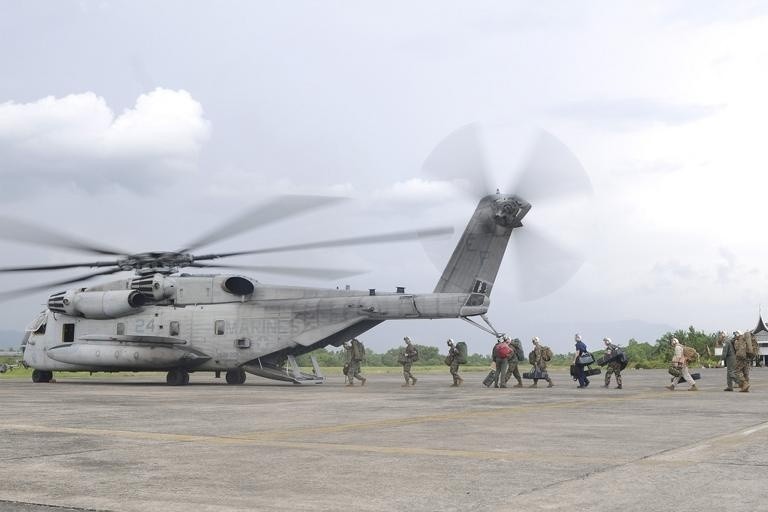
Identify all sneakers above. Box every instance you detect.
[688,384,697,391]
[495,384,554,388]
[577,381,623,388]
[347,379,366,388]
[664,384,675,391]
[403,378,417,387]
[726,384,750,392]
[451,379,465,386]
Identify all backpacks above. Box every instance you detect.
[413,349,419,361]
[541,345,552,361]
[511,339,524,361]
[743,332,758,357]
[350,339,365,361]
[683,347,698,363]
[454,342,467,364]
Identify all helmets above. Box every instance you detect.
[670,338,678,345]
[575,334,581,342]
[533,336,539,344]
[733,330,743,337]
[404,337,410,343]
[447,339,454,346]
[604,338,611,344]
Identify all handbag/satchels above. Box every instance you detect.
[444,355,452,365]
[496,343,512,358]
[575,353,594,365]
[668,367,683,377]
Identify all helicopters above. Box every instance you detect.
[1,125,589,385]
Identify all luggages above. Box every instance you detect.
[482,370,495,386]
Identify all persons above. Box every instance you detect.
[343,338,366,387]
[446,338,464,388]
[665,338,698,391]
[400,336,417,388]
[571,334,590,388]
[717,329,751,392]
[600,338,623,389]
[492,335,523,388]
[528,337,555,388]
